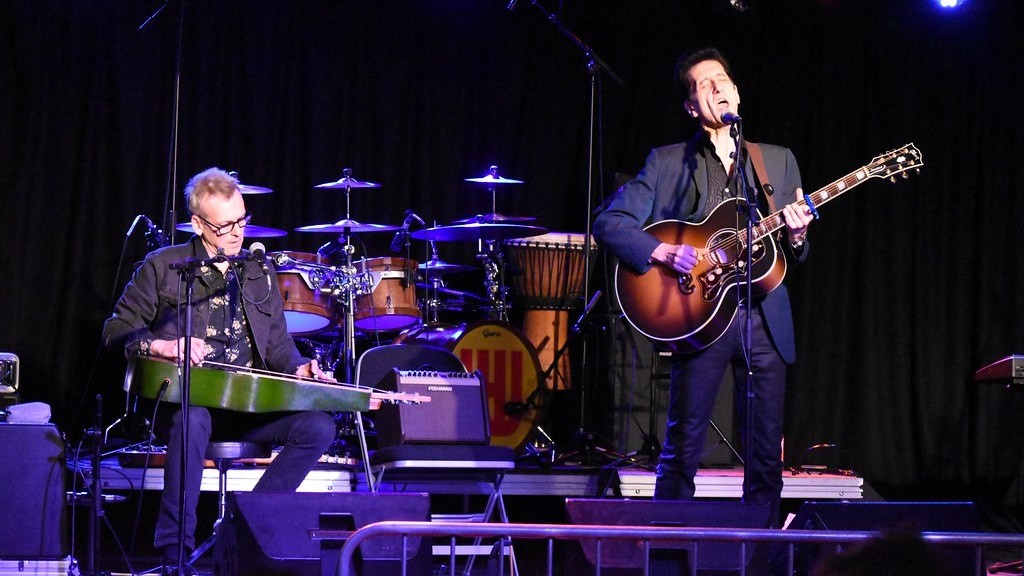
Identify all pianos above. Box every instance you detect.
[974,355,1023,388]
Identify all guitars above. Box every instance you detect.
[116,349,434,416]
[118,446,357,469]
[616,139,928,357]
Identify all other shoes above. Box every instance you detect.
[160,544,191,576]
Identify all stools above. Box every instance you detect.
[189,440,272,563]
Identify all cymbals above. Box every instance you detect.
[176,221,288,240]
[294,219,403,232]
[408,222,549,245]
[312,177,382,189]
[237,183,275,194]
[449,213,537,225]
[463,175,526,185]
[417,261,480,271]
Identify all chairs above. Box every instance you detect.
[355,344,518,576]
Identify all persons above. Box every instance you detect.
[592,47,815,529]
[101,165,338,576]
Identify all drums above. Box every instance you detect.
[506,231,599,394]
[354,254,424,333]
[391,319,544,453]
[264,250,336,337]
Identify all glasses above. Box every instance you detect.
[198,210,253,236]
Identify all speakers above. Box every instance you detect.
[560,499,988,576]
[213,492,433,576]
[371,371,491,447]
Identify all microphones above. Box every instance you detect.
[249,242,268,274]
[141,214,169,248]
[504,402,547,416]
[389,215,412,253]
[507,0,517,11]
[318,236,347,258]
[721,109,743,124]
